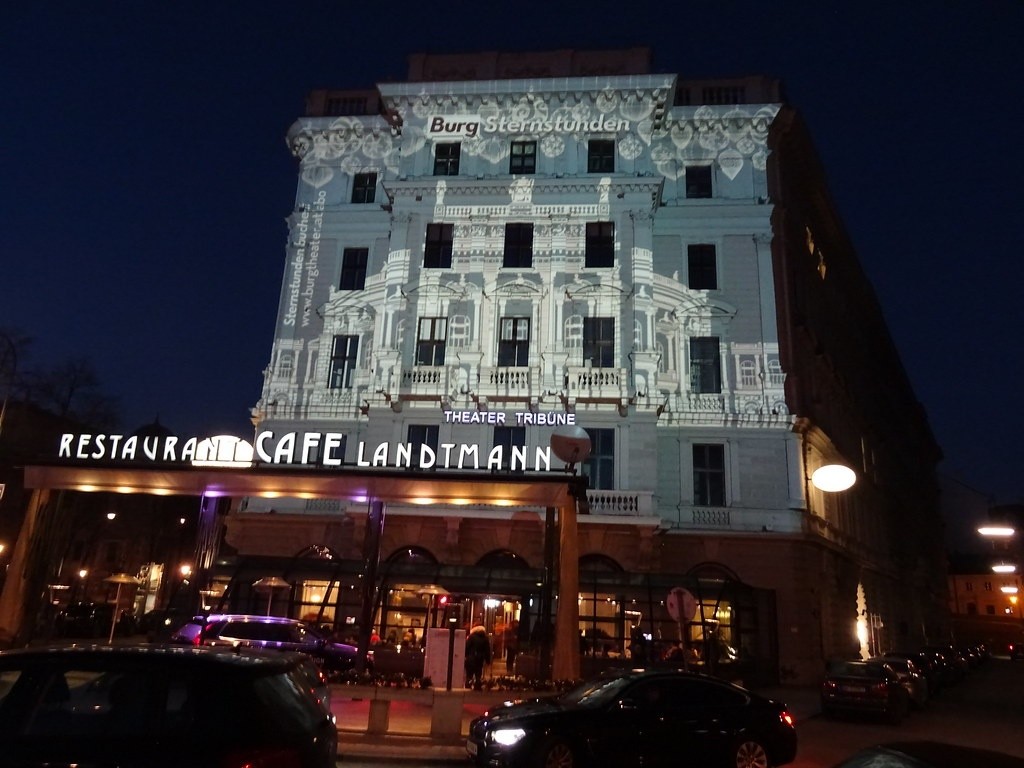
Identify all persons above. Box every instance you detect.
[493,617,508,658]
[629,626,726,666]
[386,629,416,646]
[1007,640,1018,664]
[504,621,519,674]
[839,643,862,665]
[465,626,492,690]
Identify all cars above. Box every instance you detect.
[909,640,987,677]
[822,660,913,719]
[1009,642,1024,660]
[465,671,799,768]
[872,653,927,703]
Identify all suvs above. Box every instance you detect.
[166,615,381,682]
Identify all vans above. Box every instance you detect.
[0,639,341,768]
